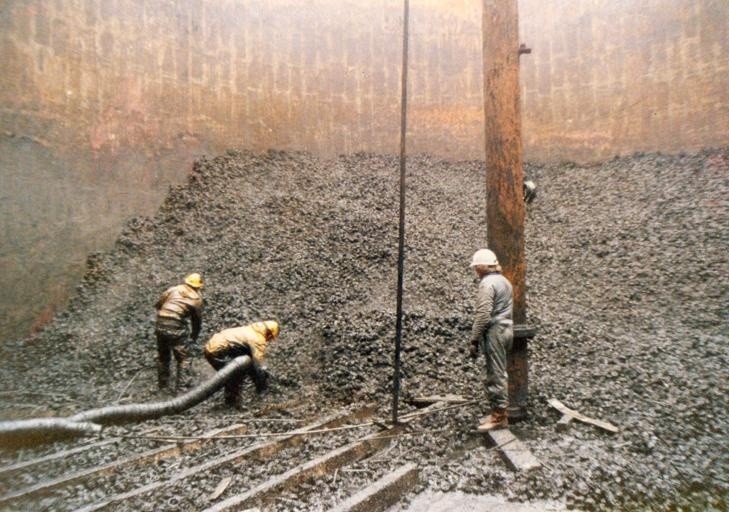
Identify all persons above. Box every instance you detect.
[464,247,514,433]
[203,319,280,412]
[154,274,206,394]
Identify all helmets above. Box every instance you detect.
[264,319,281,340]
[184,272,205,289]
[468,248,499,268]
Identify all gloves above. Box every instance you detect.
[469,340,480,363]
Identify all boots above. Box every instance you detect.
[477,408,509,433]
[478,409,495,424]
